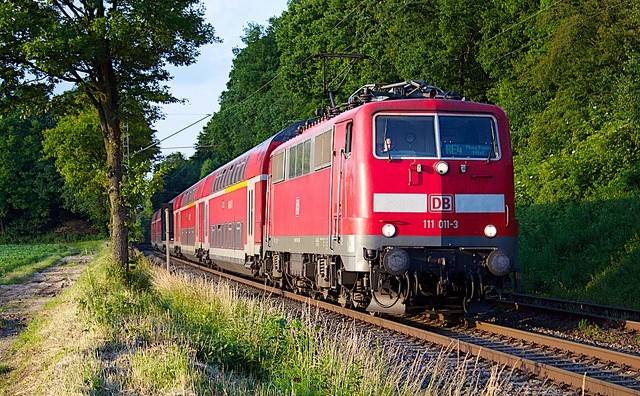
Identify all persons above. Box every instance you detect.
[383,137,392,153]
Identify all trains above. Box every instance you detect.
[150,80,519,317]
